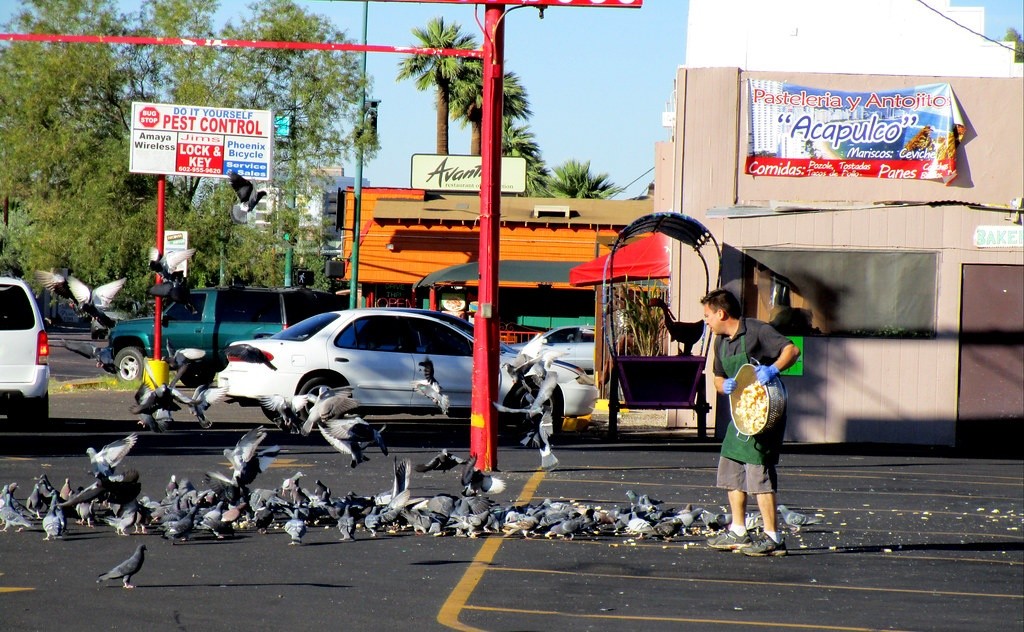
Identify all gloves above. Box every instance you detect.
[754,364,780,386]
[722,377,738,395]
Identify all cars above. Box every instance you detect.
[508,326,596,370]
[215,303,602,446]
[91,298,136,339]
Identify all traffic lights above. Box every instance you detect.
[282,214,296,244]
[297,269,315,286]
[326,186,342,231]
[366,99,380,147]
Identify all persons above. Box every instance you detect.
[701,290,800,558]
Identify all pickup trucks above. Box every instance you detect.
[98,287,347,389]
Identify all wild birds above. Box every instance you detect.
[492,332,560,472]
[409,358,450,415]
[148,246,197,279]
[257,382,388,469]
[61,339,121,375]
[146,282,199,315]
[36,269,128,329]
[129,342,277,438]
[228,169,267,226]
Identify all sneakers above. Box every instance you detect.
[707,526,752,549]
[745,531,788,556]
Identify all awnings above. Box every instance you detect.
[571,233,672,284]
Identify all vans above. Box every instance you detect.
[0,278,53,429]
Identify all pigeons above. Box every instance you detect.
[776,504,822,533]
[0,432,775,543]
[98,544,147,588]
